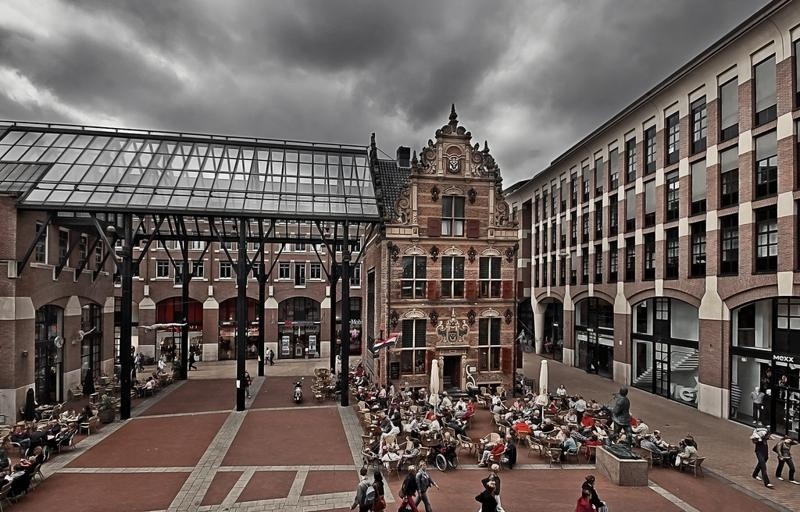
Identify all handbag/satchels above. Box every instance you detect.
[373,495,386,511]
[772,442,783,453]
[399,488,406,498]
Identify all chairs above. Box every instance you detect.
[474,393,708,481]
[1,366,171,512]
[346,367,473,482]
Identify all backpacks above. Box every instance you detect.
[362,481,376,506]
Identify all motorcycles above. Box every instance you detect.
[292,377,305,404]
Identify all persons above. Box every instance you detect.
[264,347,269,366]
[352,372,476,470]
[490,383,649,455]
[610,386,632,449]
[576,475,604,511]
[640,430,698,468]
[479,434,517,466]
[750,386,766,427]
[398,460,440,511]
[752,427,774,490]
[269,349,274,366]
[351,468,384,511]
[776,438,799,485]
[244,370,251,399]
[0,334,197,499]
[475,464,503,511]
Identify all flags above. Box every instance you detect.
[373,334,398,350]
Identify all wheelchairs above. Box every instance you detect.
[24,437,53,462]
[427,444,459,471]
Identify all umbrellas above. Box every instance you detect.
[536,359,549,420]
[428,359,440,416]
